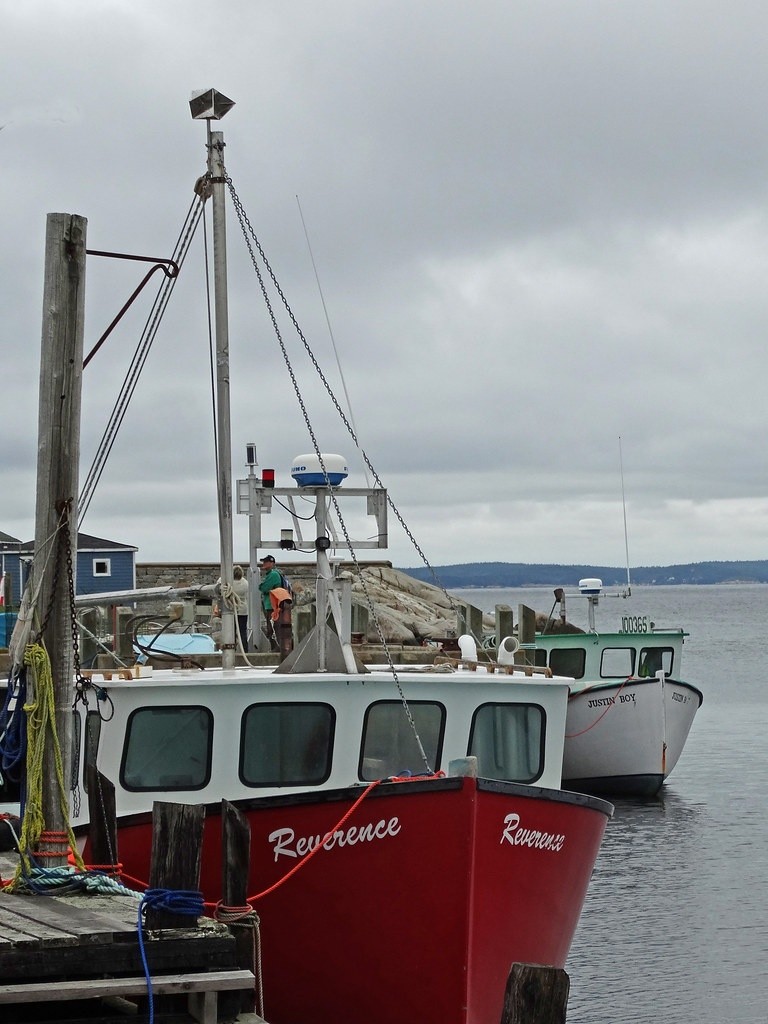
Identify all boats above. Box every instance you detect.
[479,434,704,801]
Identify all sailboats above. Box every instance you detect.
[2,88,614,1024]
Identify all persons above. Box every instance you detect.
[259,554,283,651]
[233,564,249,653]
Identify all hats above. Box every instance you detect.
[260,555,276,563]
